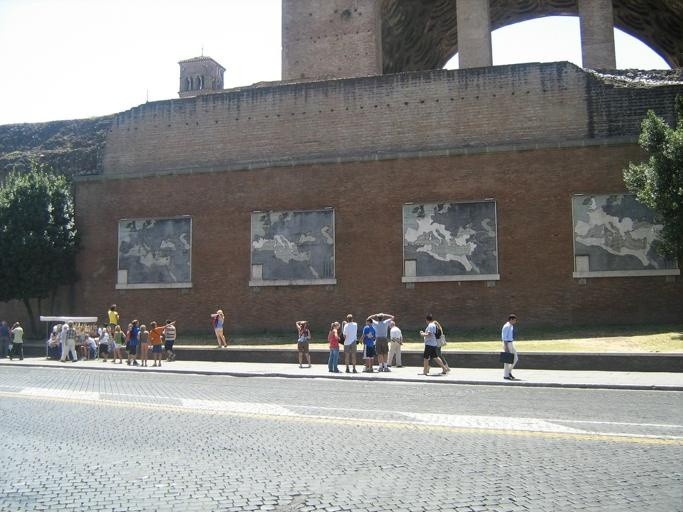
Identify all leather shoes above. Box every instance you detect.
[505,376,512,380]
[510,374,514,379]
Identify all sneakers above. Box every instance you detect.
[329,368,391,373]
[442,368,449,374]
[103,359,162,367]
[417,371,427,375]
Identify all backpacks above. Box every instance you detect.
[434,325,441,339]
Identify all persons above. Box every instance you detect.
[327,312,451,375]
[47,304,176,367]
[502,313,518,380]
[211,310,227,349]
[0,321,25,360]
[295,320,312,368]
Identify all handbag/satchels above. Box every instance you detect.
[440,335,446,347]
